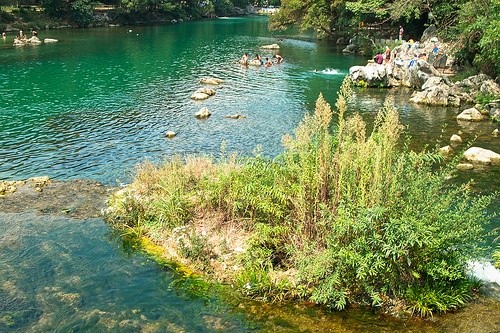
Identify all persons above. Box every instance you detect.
[32,30,38,36]
[432,45,438,57]
[373,52,383,64]
[394,49,399,57]
[255,54,261,61]
[274,54,284,63]
[314,69,318,71]
[242,52,249,60]
[2,31,6,37]
[408,38,414,45]
[16,36,18,37]
[386,47,390,62]
[264,57,272,66]
[399,25,403,41]
